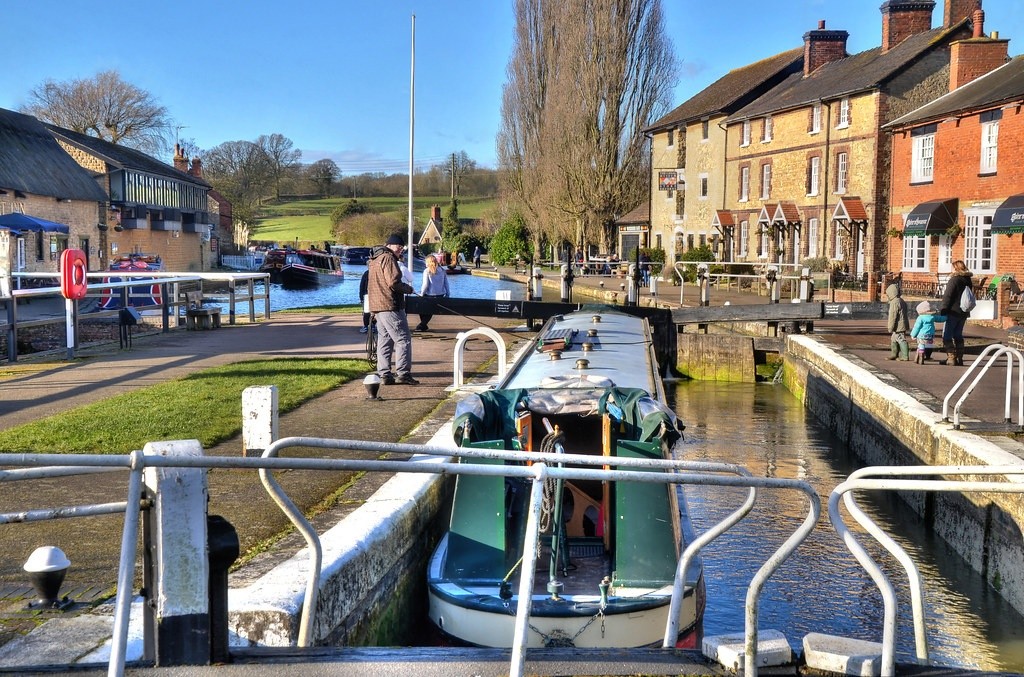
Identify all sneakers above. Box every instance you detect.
[381,377,395,385]
[395,373,419,384]
[359,326,368,332]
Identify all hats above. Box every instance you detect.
[387,234,405,247]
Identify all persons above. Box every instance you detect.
[559,249,583,267]
[324,241,332,255]
[886,283,910,361]
[939,260,973,366]
[367,236,420,385]
[910,301,937,360]
[474,246,481,268]
[414,255,451,332]
[309,245,317,250]
[639,251,652,288]
[601,254,620,274]
[359,260,375,333]
[397,254,414,285]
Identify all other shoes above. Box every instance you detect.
[415,325,429,332]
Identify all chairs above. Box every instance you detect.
[832,271,867,292]
[1010,281,1024,309]
[972,277,990,301]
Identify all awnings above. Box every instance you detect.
[990,195,1024,234]
[903,198,958,236]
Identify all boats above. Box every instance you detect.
[258,237,462,289]
[419,302,708,648]
[94,246,174,316]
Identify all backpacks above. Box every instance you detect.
[952,280,975,313]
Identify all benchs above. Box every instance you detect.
[612,260,631,276]
[185,289,223,329]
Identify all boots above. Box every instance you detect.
[896,343,909,361]
[885,342,899,361]
[957,349,963,365]
[939,349,958,365]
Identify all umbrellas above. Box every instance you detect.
[0,212,69,290]
